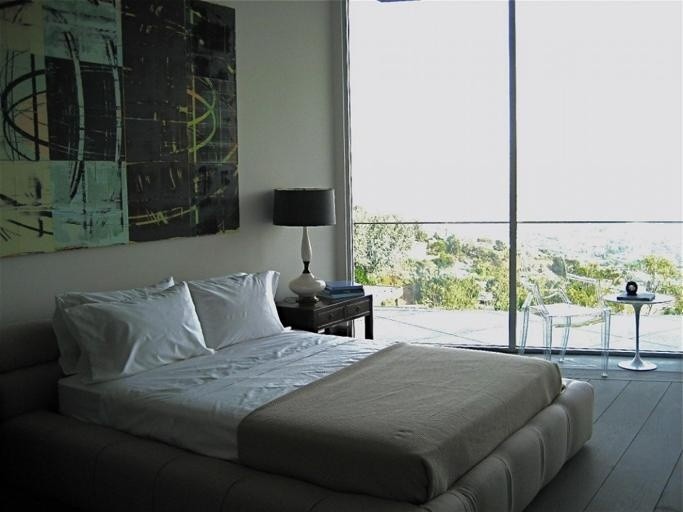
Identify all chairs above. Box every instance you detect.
[517,276,613,379]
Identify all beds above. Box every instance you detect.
[0,319,592,512]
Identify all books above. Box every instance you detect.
[616,293,655,302]
[318,279,365,300]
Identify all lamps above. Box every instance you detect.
[272,187,336,304]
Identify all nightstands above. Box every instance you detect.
[275,293,373,339]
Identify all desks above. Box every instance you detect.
[603,291,675,371]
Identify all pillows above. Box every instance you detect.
[48,269,288,384]
